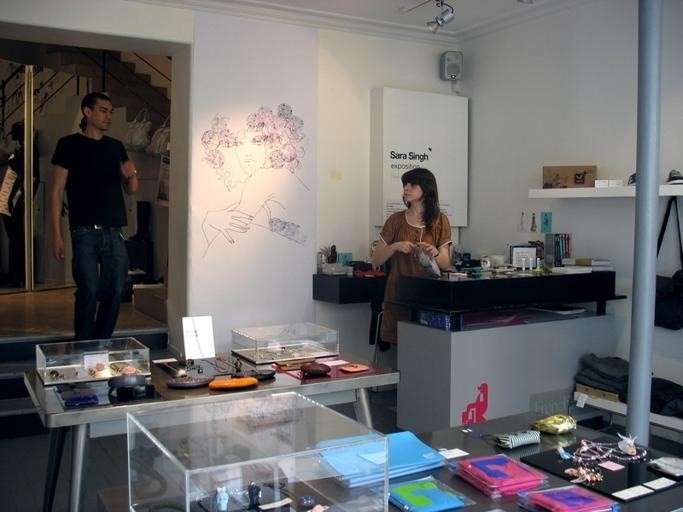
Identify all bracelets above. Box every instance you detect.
[434,252,439,258]
[128,169,137,178]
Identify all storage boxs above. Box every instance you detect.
[542,164,598,189]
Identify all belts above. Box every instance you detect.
[71,224,121,229]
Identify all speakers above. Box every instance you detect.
[440,51,463,80]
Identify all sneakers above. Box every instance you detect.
[629,173,636,186]
[667,170,683,184]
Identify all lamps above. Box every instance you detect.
[425,1,456,35]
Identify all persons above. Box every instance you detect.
[1,119,40,287]
[51,90,139,341]
[201,102,313,256]
[370,167,455,348]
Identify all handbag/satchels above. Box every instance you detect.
[146,116,170,157]
[655,268,683,330]
[124,108,152,152]
[534,414,576,435]
[496,430,540,449]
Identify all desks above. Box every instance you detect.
[22,351,400,508]
[92,415,681,511]
[570,372,683,434]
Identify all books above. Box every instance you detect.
[544,232,612,275]
[527,304,586,315]
[312,431,447,491]
